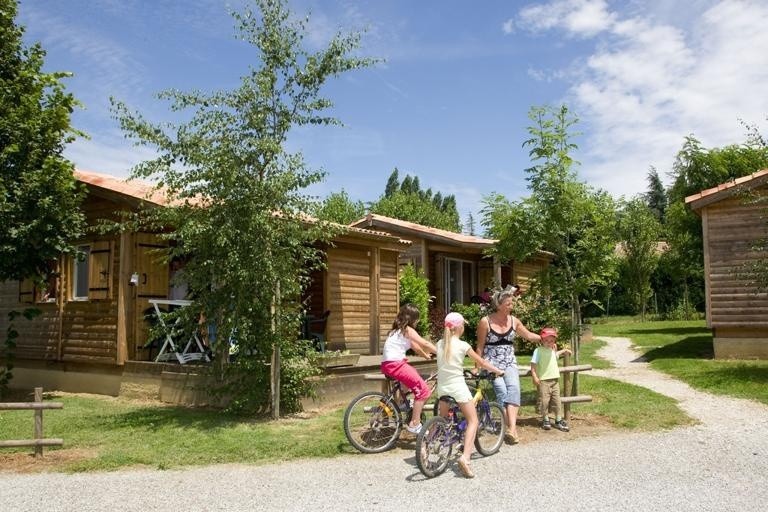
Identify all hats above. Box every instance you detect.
[445,311,468,330]
[540,326,558,339]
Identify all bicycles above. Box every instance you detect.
[342,349,509,480]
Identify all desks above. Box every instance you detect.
[147,297,212,365]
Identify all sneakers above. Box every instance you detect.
[554,419,570,432]
[541,420,551,430]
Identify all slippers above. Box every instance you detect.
[406,423,429,436]
[504,430,520,444]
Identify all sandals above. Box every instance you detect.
[421,447,433,469]
[457,455,475,478]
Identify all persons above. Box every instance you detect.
[169,256,190,301]
[481,287,491,304]
[513,285,521,297]
[470,290,557,444]
[381,303,437,437]
[530,327,571,433]
[435,312,506,478]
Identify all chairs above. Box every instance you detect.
[471,296,483,307]
[299,309,331,354]
[144,306,183,362]
